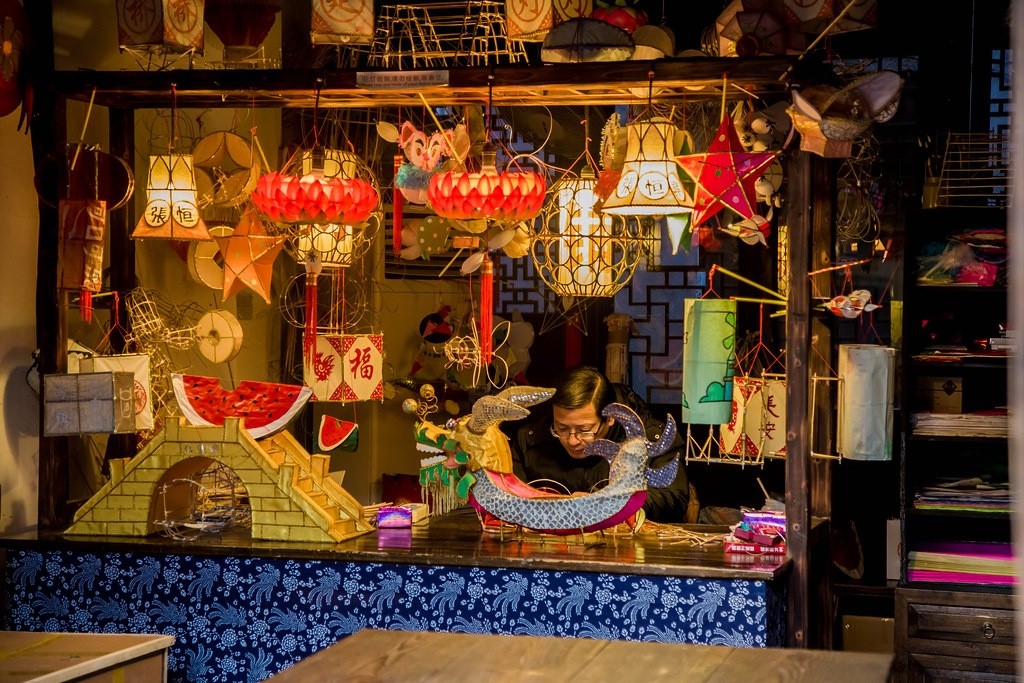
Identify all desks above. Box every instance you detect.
[0,630,176,683]
[260,630,894,683]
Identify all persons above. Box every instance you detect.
[520,364,691,528]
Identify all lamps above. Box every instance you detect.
[55,80,786,367]
[116,0,204,57]
[310,0,375,48]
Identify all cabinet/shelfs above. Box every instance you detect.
[900,208,1023,595]
[895,584,1023,683]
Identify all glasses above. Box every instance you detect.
[550,419,603,439]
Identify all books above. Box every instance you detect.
[909,410,1008,439]
[906,542,1014,585]
[885,516,902,581]
[917,329,1024,353]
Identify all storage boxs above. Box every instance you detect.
[930,376,962,414]
[842,615,895,653]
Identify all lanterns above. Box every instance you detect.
[719,371,770,458]
[763,379,788,460]
[0,0,909,542]
